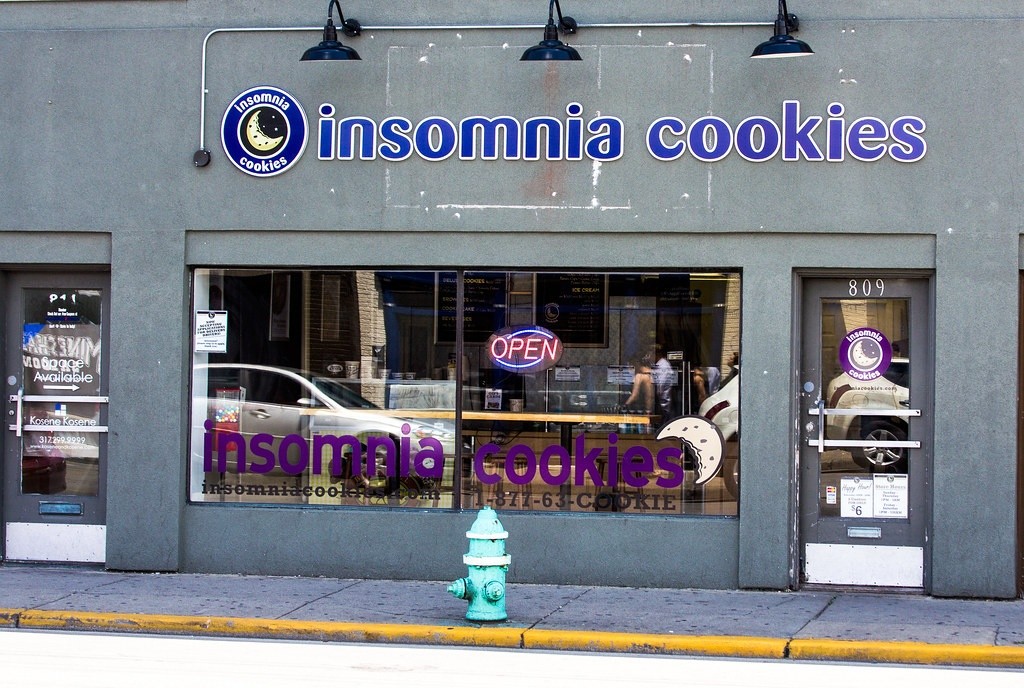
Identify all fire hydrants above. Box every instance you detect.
[446,506,511,622]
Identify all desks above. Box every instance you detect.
[299,407,650,514]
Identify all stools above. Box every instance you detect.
[472,454,533,511]
[342,450,392,506]
[593,453,645,509]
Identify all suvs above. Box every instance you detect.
[23,420,67,495]
[696,373,739,500]
[826,357,911,470]
[192,361,455,495]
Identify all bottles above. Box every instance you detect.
[619,410,628,434]
[628,410,636,434]
[644,410,653,434]
[636,410,644,434]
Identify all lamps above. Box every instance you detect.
[519,0,584,61]
[299,0,363,62]
[749,0,815,59]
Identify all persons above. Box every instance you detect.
[621,344,740,434]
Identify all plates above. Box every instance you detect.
[556,429,586,433]
[588,429,617,434]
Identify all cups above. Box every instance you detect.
[327,363,343,377]
[392,373,404,380]
[378,369,391,379]
[405,373,416,380]
[345,361,360,378]
